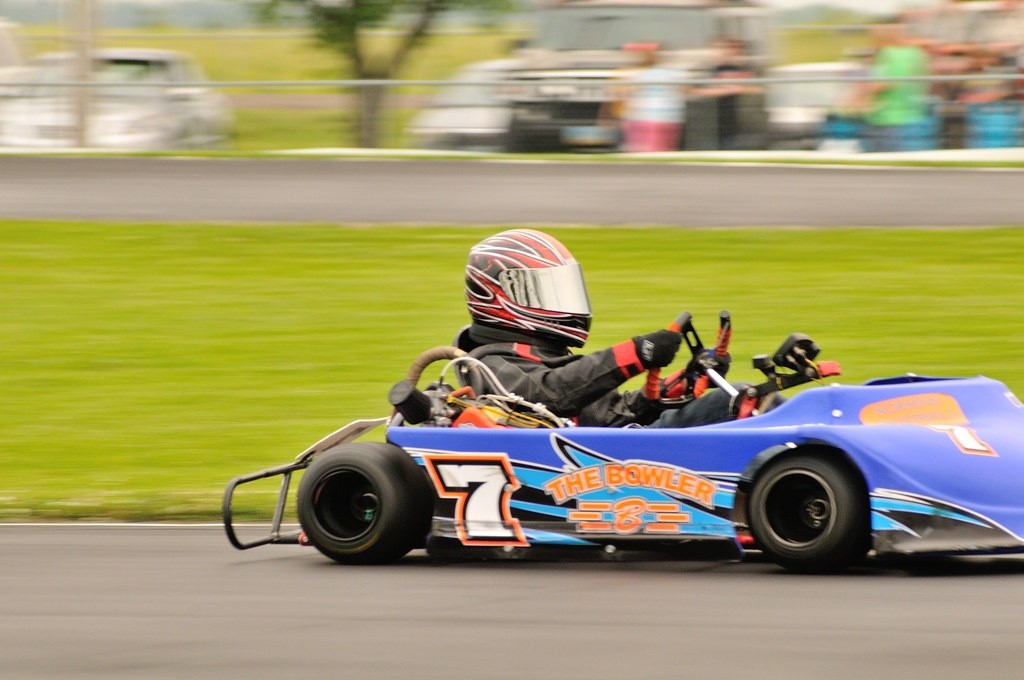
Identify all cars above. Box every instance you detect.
[0,45,232,152]
[760,58,868,147]
[411,51,527,147]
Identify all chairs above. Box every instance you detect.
[452,328,474,389]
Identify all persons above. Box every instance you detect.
[461,228,787,427]
[490,16,1021,153]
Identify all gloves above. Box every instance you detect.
[630,328,682,369]
[685,357,731,389]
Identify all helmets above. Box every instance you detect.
[463,228,594,349]
